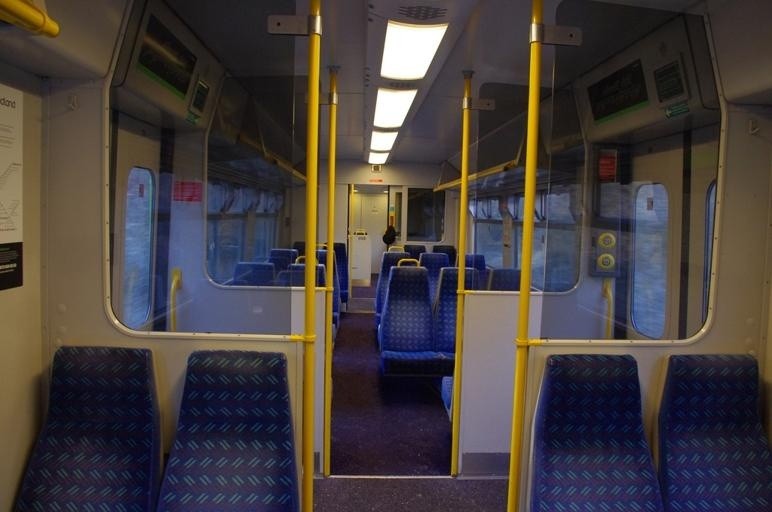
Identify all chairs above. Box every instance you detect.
[232,239,350,349]
[10,339,166,511]
[656,351,772,512]
[374,243,522,378]
[518,351,665,512]
[155,345,304,510]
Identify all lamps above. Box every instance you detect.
[357,1,470,179]
